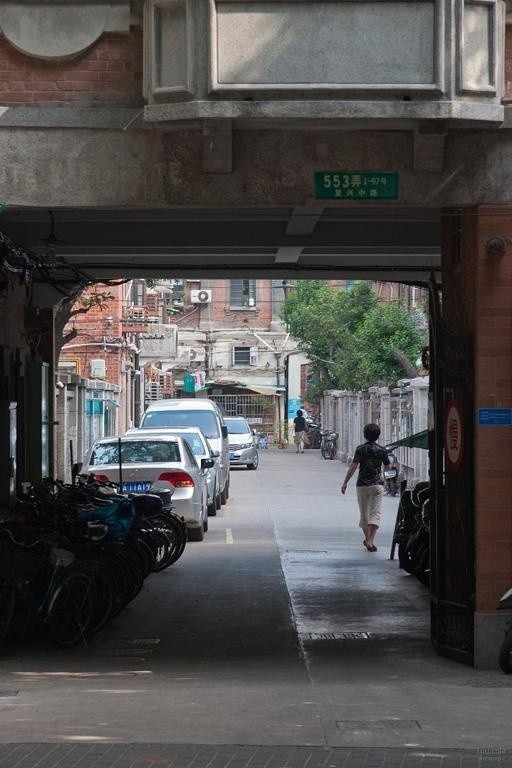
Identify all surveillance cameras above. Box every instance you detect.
[105,317,113,322]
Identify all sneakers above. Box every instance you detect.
[362,539,378,553]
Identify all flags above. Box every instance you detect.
[182,369,206,393]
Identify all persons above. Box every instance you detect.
[298,405,308,418]
[340,423,392,552]
[291,409,307,453]
